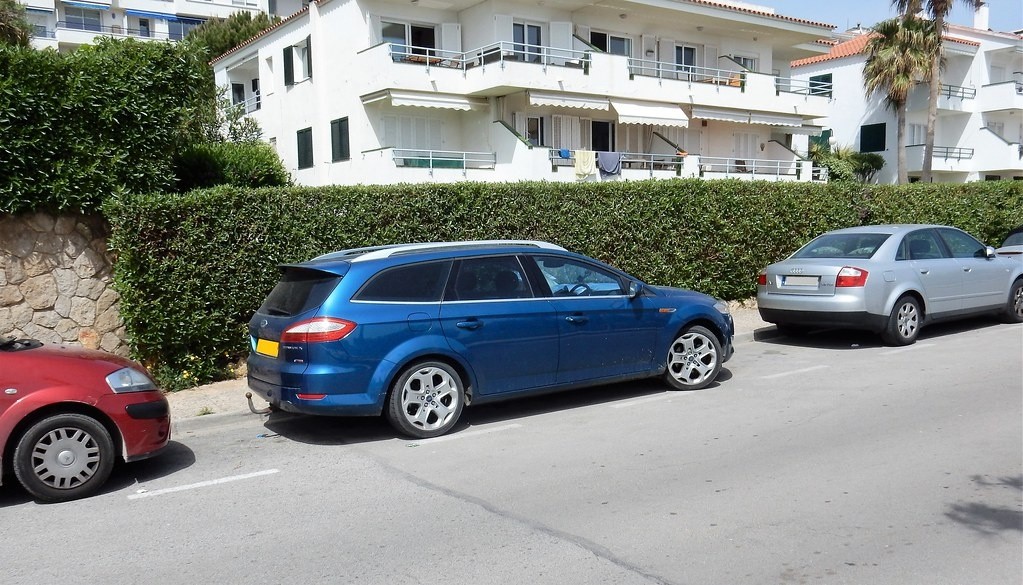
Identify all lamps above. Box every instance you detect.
[646,49,654,58]
[760,143,764,151]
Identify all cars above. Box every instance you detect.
[246,238,736,438]
[996,226,1023,256]
[0,337,173,505]
[756,224,1023,347]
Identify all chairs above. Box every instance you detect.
[736,160,756,174]
[495,272,522,300]
[458,273,480,295]
[911,240,934,258]
